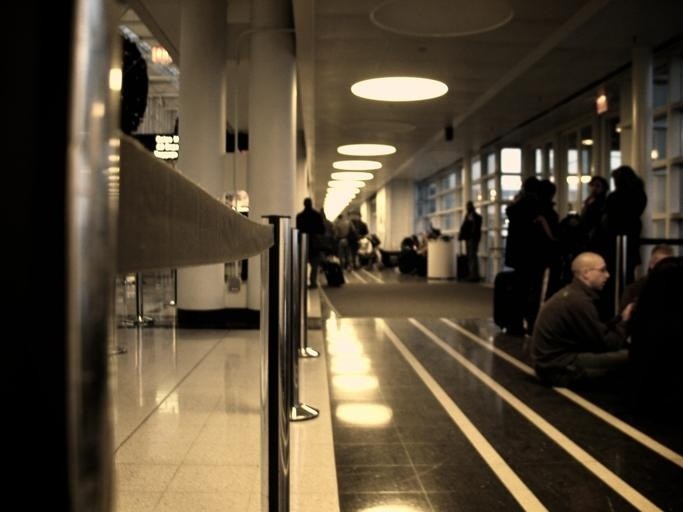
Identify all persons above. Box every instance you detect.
[296,197,483,288]
[495,167,681,430]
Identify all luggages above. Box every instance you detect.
[325,262,345,287]
[457,240,469,282]
[493,271,514,331]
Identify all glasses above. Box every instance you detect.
[589,266,607,272]
